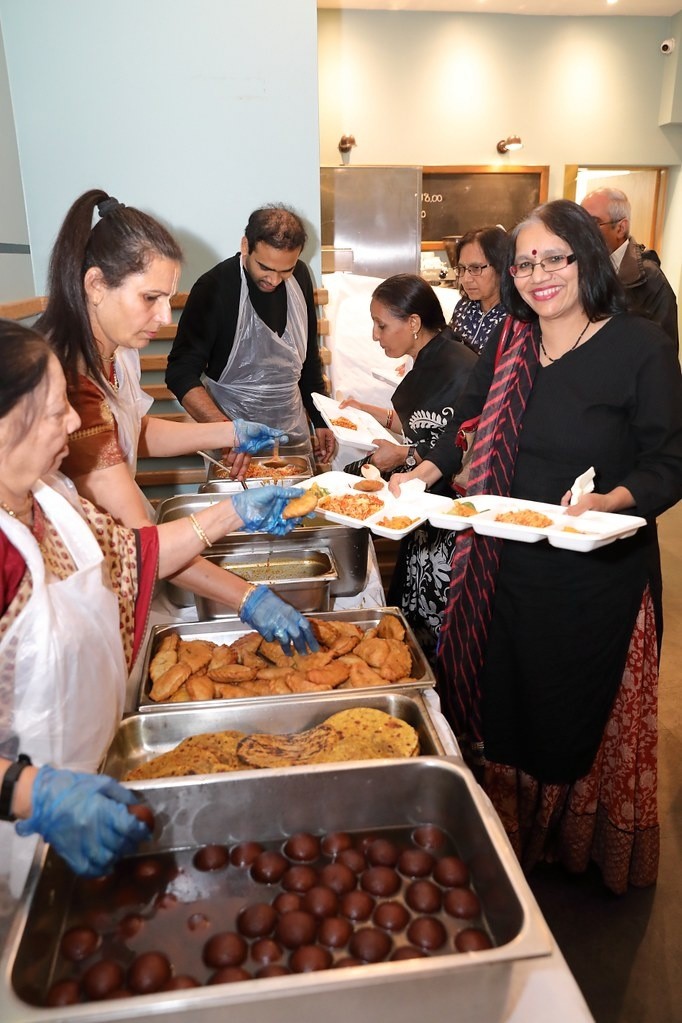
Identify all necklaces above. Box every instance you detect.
[537,319,590,363]
[0,492,35,515]
[93,349,119,393]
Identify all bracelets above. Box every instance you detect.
[189,512,212,548]
[385,408,394,429]
[238,583,255,618]
[0,753,32,823]
[233,419,238,448]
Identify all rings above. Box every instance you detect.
[275,629,284,637]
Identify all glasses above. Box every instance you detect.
[593,217,626,230]
[509,254,577,277]
[453,264,490,277]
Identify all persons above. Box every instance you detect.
[387,197,682,896]
[338,270,479,635]
[0,320,317,883]
[24,190,323,660]
[163,202,336,484]
[578,186,680,360]
[446,222,510,355]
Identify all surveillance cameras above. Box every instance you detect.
[661,39,674,54]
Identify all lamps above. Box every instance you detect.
[339,135,356,153]
[496,135,522,153]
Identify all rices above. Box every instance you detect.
[321,492,386,521]
[495,508,553,528]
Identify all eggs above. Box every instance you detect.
[128,804,154,831]
[44,826,492,1010]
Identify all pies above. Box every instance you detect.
[353,479,385,492]
[282,492,318,518]
[150,615,418,704]
[119,708,421,783]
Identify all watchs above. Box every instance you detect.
[405,446,417,467]
